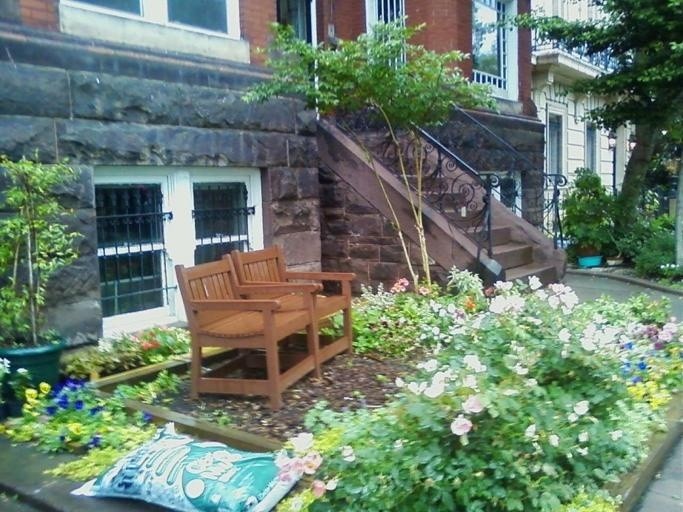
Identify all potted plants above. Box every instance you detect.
[564,168,605,267]
[0,154,87,399]
[605,190,632,266]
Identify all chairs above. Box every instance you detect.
[175,255,319,410]
[230,245,356,361]
